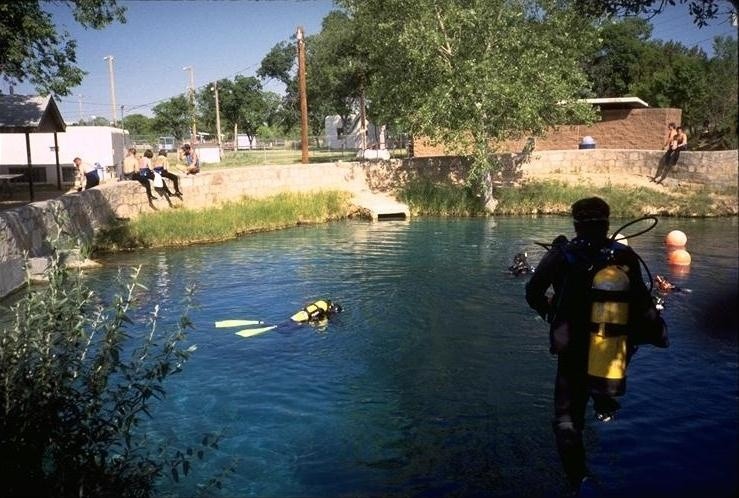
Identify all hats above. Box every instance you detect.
[181,144,190,148]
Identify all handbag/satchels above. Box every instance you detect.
[152,173,164,188]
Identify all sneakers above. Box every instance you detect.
[149,190,184,200]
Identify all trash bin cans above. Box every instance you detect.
[579,136,596,149]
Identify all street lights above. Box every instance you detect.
[182,66,223,157]
[295,27,309,164]
[103,55,117,127]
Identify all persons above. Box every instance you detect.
[63,156,104,196]
[661,122,677,149]
[664,127,689,166]
[523,196,669,498]
[215,299,342,338]
[502,251,533,277]
[115,143,200,200]
[640,271,693,305]
